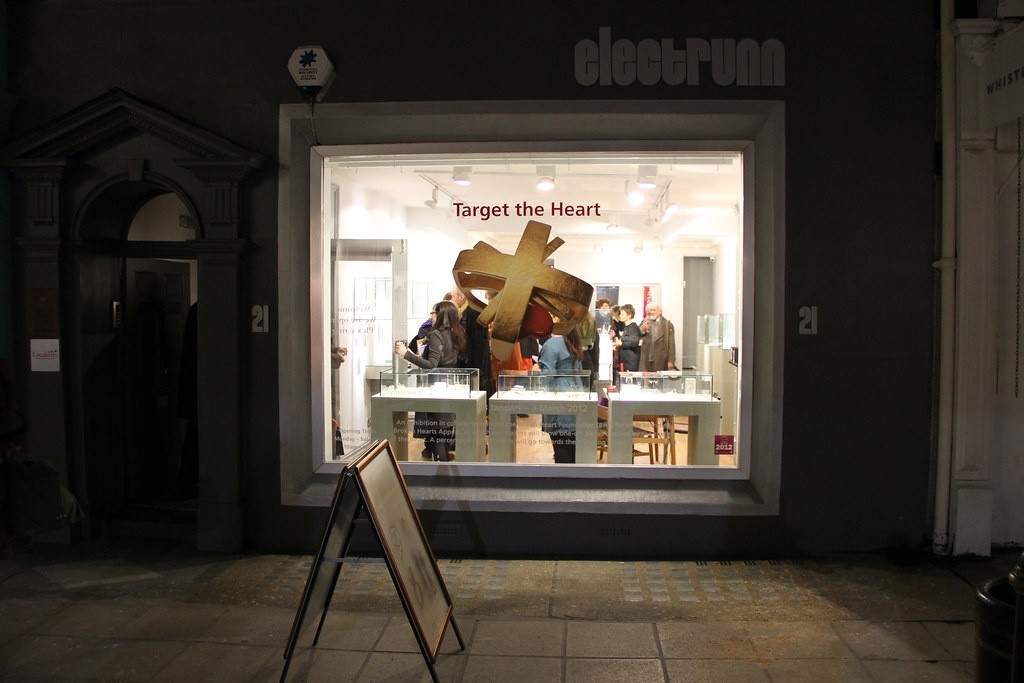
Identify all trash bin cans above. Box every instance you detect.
[972,575,1024,683]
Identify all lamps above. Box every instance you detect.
[646,186,679,231]
[452,166,473,185]
[535,166,556,190]
[447,197,469,225]
[424,186,439,209]
[637,165,658,190]
[624,179,644,209]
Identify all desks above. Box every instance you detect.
[487,391,598,464]
[371,387,487,463]
[598,404,677,465]
[608,392,721,465]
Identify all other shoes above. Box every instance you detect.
[421,449,433,459]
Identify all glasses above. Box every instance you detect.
[430,311,437,316]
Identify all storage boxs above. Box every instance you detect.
[614,370,713,401]
[498,369,592,399]
[696,313,735,349]
[380,368,481,398]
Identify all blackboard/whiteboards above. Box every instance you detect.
[356,439,463,659]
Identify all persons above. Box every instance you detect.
[532,312,600,464]
[397,284,539,463]
[170,301,199,503]
[596,298,676,372]
[331,345,348,369]
